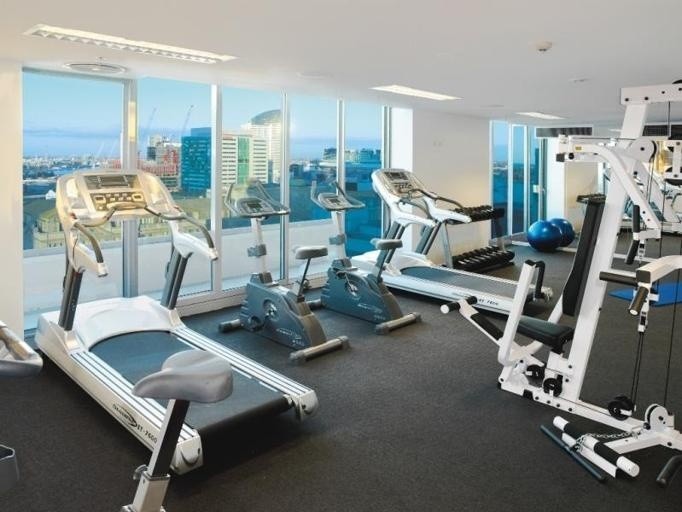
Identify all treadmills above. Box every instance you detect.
[351,168,554,316]
[35,168,319,475]
